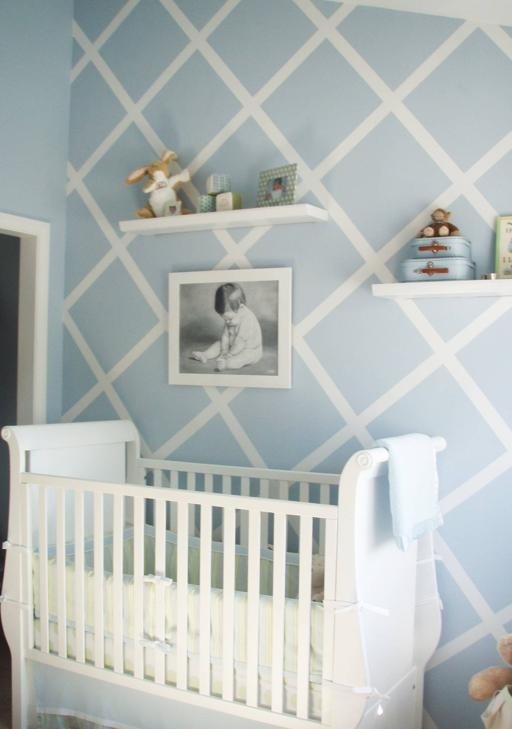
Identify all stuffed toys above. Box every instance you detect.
[465,635,512,729]
[124,152,194,219]
[416,208,459,239]
[296,554,325,603]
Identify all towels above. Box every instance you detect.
[368,430,447,552]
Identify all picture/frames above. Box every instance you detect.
[167,266,295,389]
[256,162,299,205]
[492,213,512,281]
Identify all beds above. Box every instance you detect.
[3,415,450,728]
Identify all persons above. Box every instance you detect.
[190,282,262,371]
[264,177,287,202]
